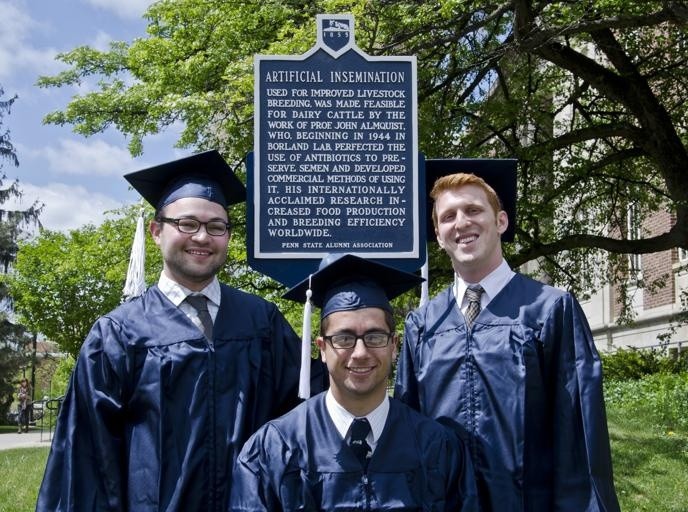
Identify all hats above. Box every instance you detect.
[422,156,518,246]
[122,150,246,214]
[277,253,429,320]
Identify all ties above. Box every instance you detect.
[182,294,222,342]
[464,284,484,327]
[349,417,376,467]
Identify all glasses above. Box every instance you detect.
[318,331,397,351]
[154,216,232,238]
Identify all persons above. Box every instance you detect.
[228,254,481,511]
[36,151,327,512]
[393,157,621,511]
[17,377,33,434]
